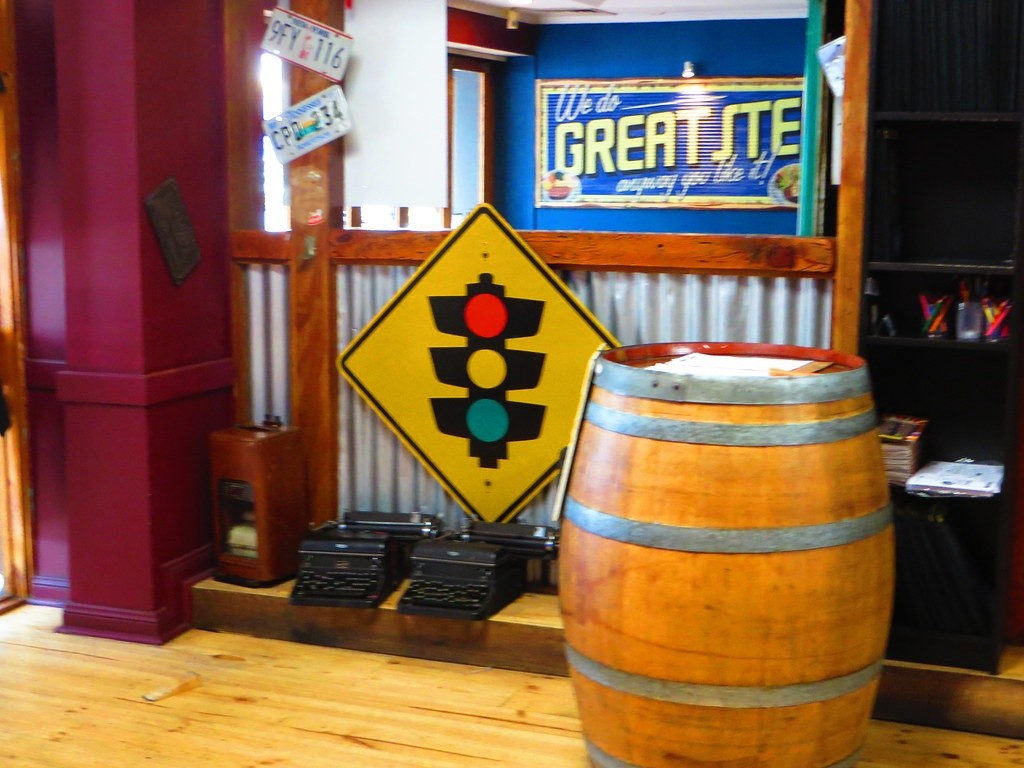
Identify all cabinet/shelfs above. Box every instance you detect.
[858,0,1024,679]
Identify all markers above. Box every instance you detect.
[919,293,953,339]
[958,278,1016,339]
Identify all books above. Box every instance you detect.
[878,415,929,487]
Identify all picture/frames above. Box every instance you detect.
[535,77,804,210]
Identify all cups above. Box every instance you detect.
[956,302,982,340]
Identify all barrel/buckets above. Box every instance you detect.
[559,342,894,768]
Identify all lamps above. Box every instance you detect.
[505,10,520,30]
[682,61,696,78]
